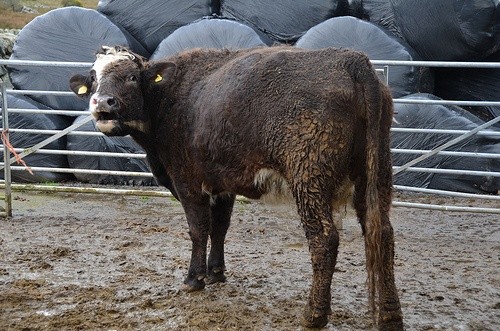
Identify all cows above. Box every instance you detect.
[70,44,404,331]
